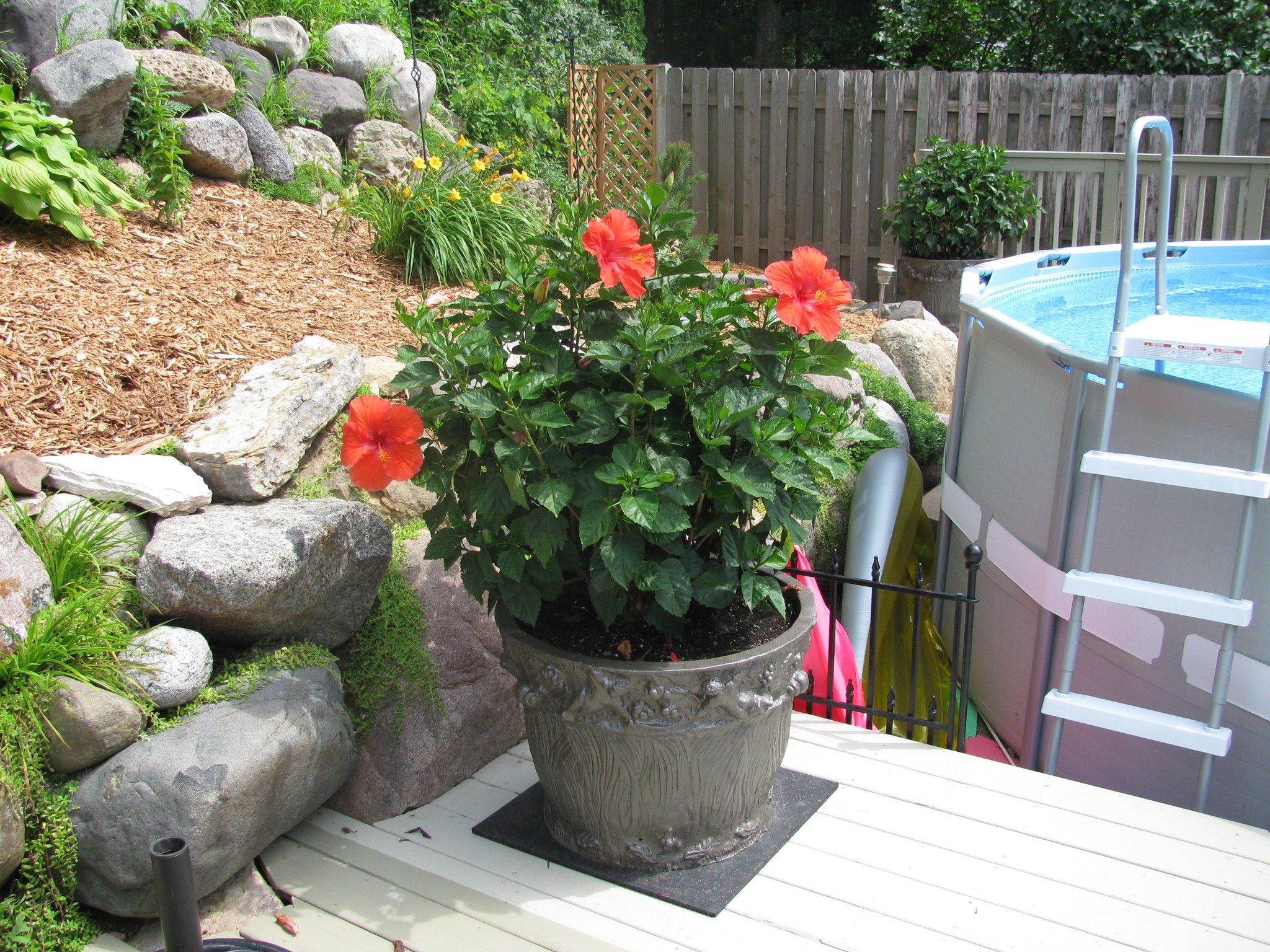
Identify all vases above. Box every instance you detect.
[495,560,816,870]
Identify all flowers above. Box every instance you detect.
[342,181,894,650]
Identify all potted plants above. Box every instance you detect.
[875,134,1048,337]
